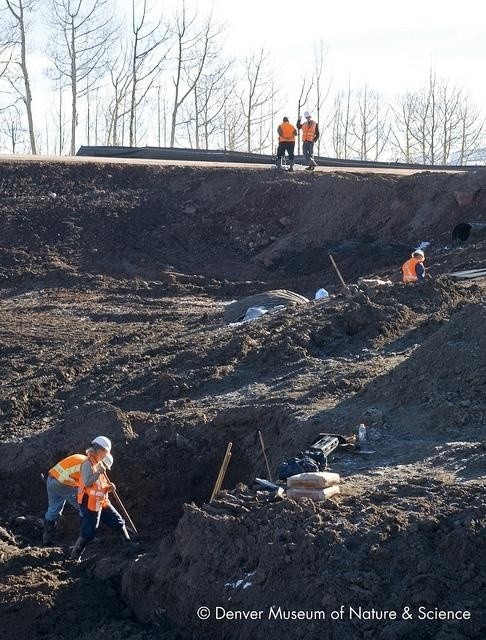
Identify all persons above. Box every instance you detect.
[275,116,298,172]
[41,445,113,547]
[401,249,428,283]
[67,433,146,577]
[297,111,319,170]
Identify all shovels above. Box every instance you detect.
[99,463,151,544]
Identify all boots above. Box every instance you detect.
[276,158,283,169]
[112,524,142,553]
[287,159,294,172]
[70,536,88,563]
[42,520,58,546]
[303,154,317,170]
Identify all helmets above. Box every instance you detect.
[102,452,115,472]
[411,249,426,262]
[90,435,113,455]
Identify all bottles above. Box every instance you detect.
[359,423,367,442]
